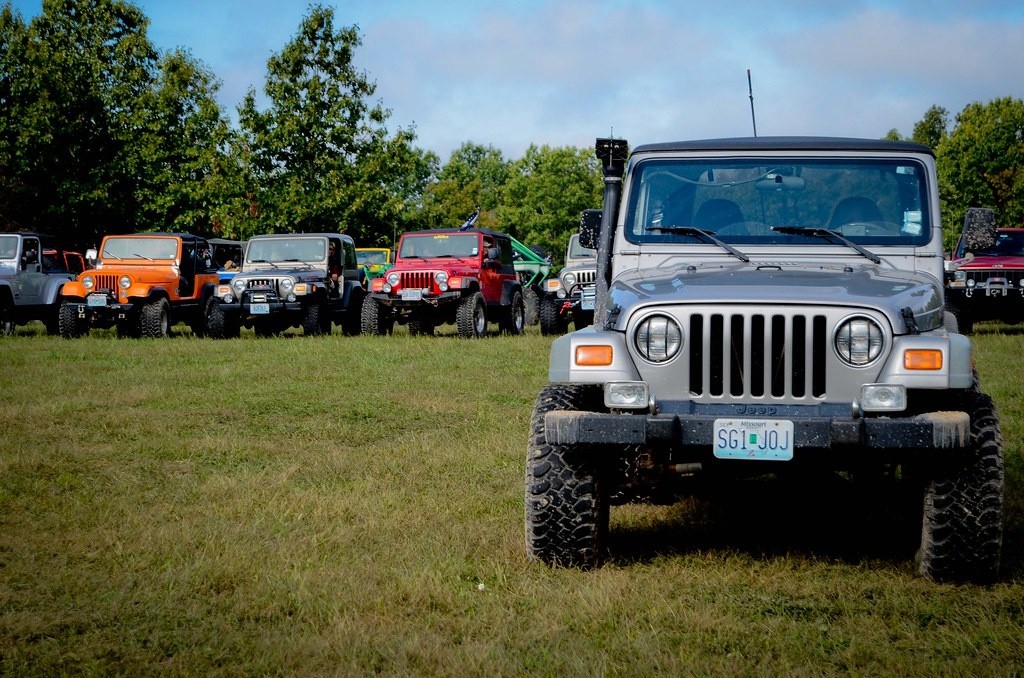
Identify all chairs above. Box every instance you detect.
[694,199,750,236]
[828,195,887,231]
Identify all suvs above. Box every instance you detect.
[943,229,1024,335]
[523,74,1003,588]
[31,248,85,281]
[505,234,554,326]
[58,234,219,338]
[190,238,247,287]
[0,230,72,336]
[531,233,597,337]
[354,247,398,289]
[209,230,369,340]
[360,206,526,339]
